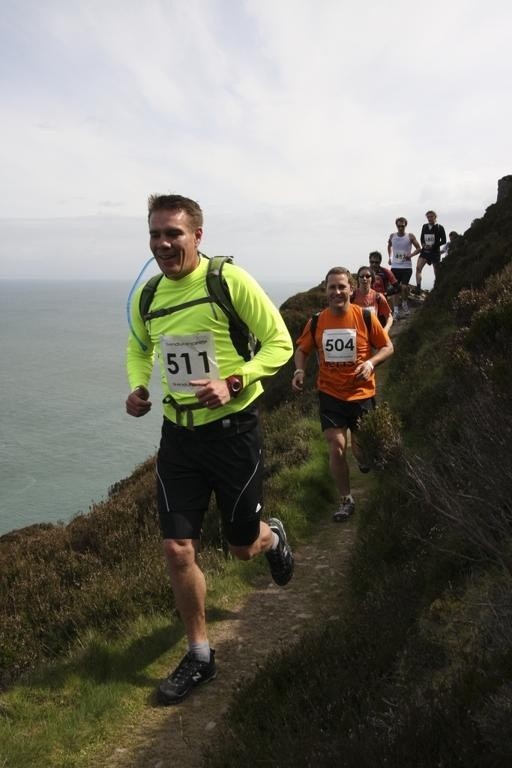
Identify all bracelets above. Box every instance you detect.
[293,368,304,376]
[365,359,375,371]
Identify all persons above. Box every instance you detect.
[387,216,422,322]
[123,193,296,707]
[440,230,458,256]
[292,266,395,522]
[350,266,394,334]
[369,250,402,300]
[414,210,446,295]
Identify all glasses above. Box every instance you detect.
[359,275,371,278]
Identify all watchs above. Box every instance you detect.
[225,374,243,398]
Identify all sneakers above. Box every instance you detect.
[266,518,295,586]
[334,497,355,521]
[158,649,217,705]
